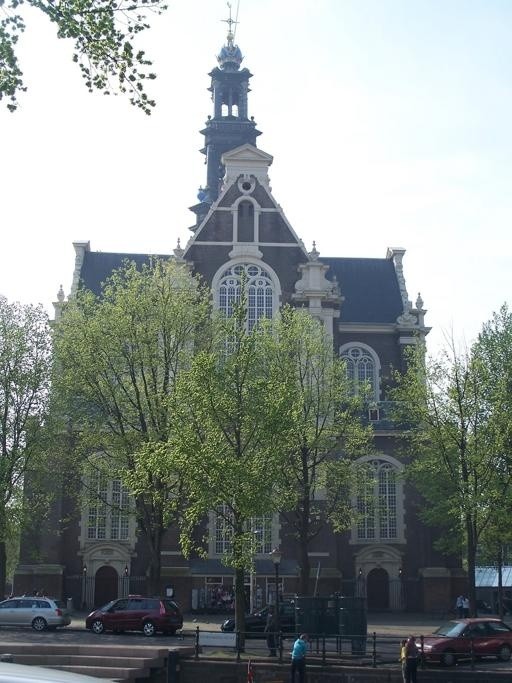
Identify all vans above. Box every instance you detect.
[86,595,183,636]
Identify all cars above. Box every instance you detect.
[221,600,296,639]
[405,617,512,666]
[0,597,71,631]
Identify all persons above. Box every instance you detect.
[461,594,471,619]
[398,639,407,683]
[455,593,462,618]
[403,634,420,682]
[288,633,311,682]
[265,607,283,656]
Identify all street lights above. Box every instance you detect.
[269,547,284,649]
